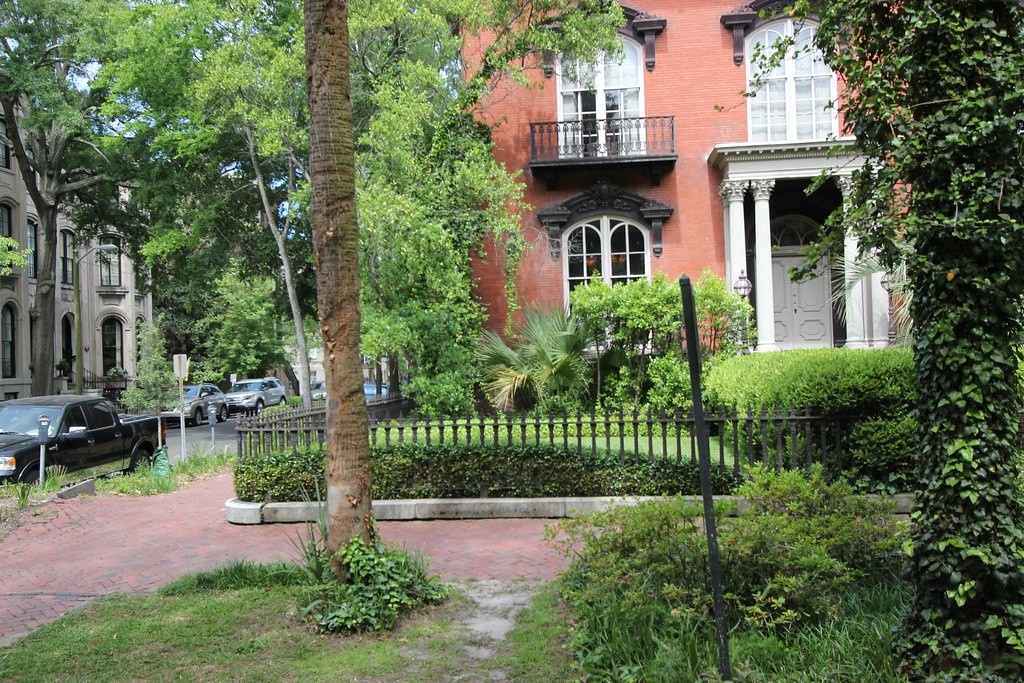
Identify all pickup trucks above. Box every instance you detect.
[0,394,168,492]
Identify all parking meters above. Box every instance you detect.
[36,413,50,490]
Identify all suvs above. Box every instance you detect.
[224,375,287,413]
[158,383,229,427]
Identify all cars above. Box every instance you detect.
[312,381,328,400]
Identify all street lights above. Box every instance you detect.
[880,270,901,342]
[733,266,751,355]
